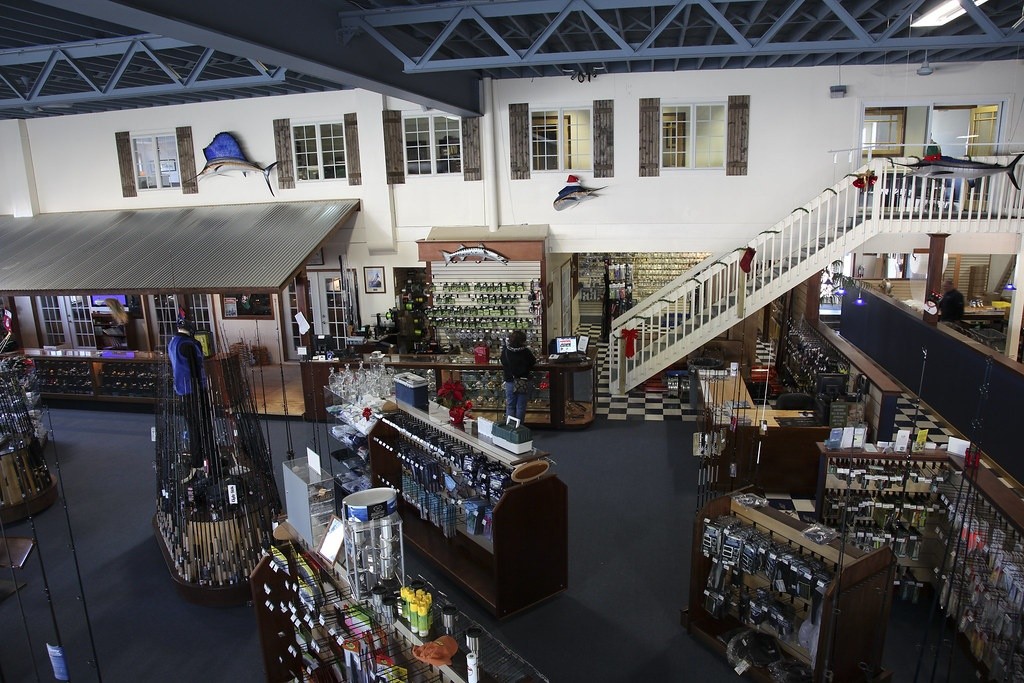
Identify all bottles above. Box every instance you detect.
[327,361,397,401]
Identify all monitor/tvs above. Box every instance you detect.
[556,336,577,359]
[312,334,334,355]
[817,373,846,403]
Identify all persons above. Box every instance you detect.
[927,280,964,323]
[500,331,536,425]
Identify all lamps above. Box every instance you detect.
[829,85,848,99]
[851,242,867,306]
[909,0,988,28]
[834,245,848,298]
[1001,233,1017,290]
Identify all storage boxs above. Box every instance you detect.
[992,301,1010,317]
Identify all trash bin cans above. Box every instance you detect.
[687,357,725,409]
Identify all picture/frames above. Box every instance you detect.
[220,292,275,320]
[364,265,387,294]
[305,247,324,266]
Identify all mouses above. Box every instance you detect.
[581,357,585,361]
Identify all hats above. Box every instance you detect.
[412,635,458,666]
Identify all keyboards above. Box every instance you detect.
[554,358,582,363]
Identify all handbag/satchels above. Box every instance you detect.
[513,376,528,394]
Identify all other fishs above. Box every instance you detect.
[438,243,508,267]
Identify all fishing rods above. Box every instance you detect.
[914,353,998,680]
[693,303,763,515]
[0,521,41,683]
[869,344,928,681]
[823,386,862,682]
[0,342,54,501]
[305,304,337,474]
[151,245,296,587]
[42,391,105,683]
[3,401,75,683]
